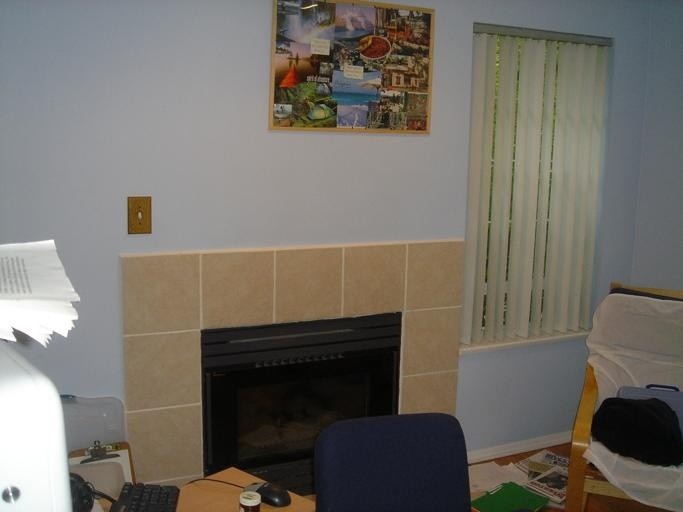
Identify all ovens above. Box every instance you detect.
[199,313,402,498]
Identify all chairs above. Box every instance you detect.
[315,410,471,512]
[565,280,682,511]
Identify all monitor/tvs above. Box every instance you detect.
[0,334,74,512]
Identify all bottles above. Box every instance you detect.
[239,491,260,512]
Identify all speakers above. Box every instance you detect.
[70,444,135,499]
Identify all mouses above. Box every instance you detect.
[243,480,291,508]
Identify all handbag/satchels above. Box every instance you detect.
[590,397,683,467]
[616,384,683,436]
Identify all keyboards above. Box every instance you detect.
[106,481,179,511]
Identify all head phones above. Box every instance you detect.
[69,466,100,512]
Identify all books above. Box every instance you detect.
[471,449,570,512]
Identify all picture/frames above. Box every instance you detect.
[267,1,436,139]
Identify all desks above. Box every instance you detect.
[99,465,315,511]
